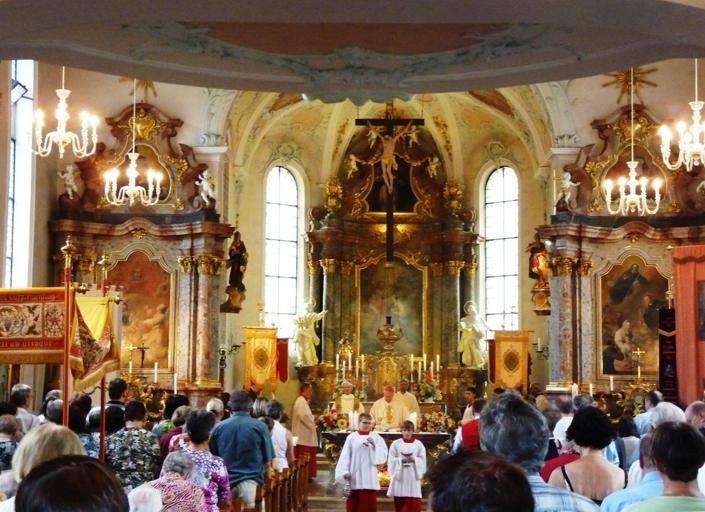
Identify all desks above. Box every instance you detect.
[321,430,450,497]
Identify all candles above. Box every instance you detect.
[536,334,543,353]
[127,361,134,372]
[610,373,616,391]
[637,365,642,379]
[173,372,178,394]
[408,351,443,379]
[333,349,366,375]
[152,362,159,383]
[588,382,597,397]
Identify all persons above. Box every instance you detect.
[459,301,489,365]
[335,413,388,512]
[367,118,413,197]
[387,421,427,512]
[1,378,318,512]
[427,386,704,511]
[331,379,420,432]
[603,264,661,374]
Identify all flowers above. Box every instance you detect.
[317,376,368,430]
[409,370,456,432]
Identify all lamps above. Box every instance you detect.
[29,64,100,161]
[103,71,164,210]
[656,58,705,174]
[602,67,664,219]
